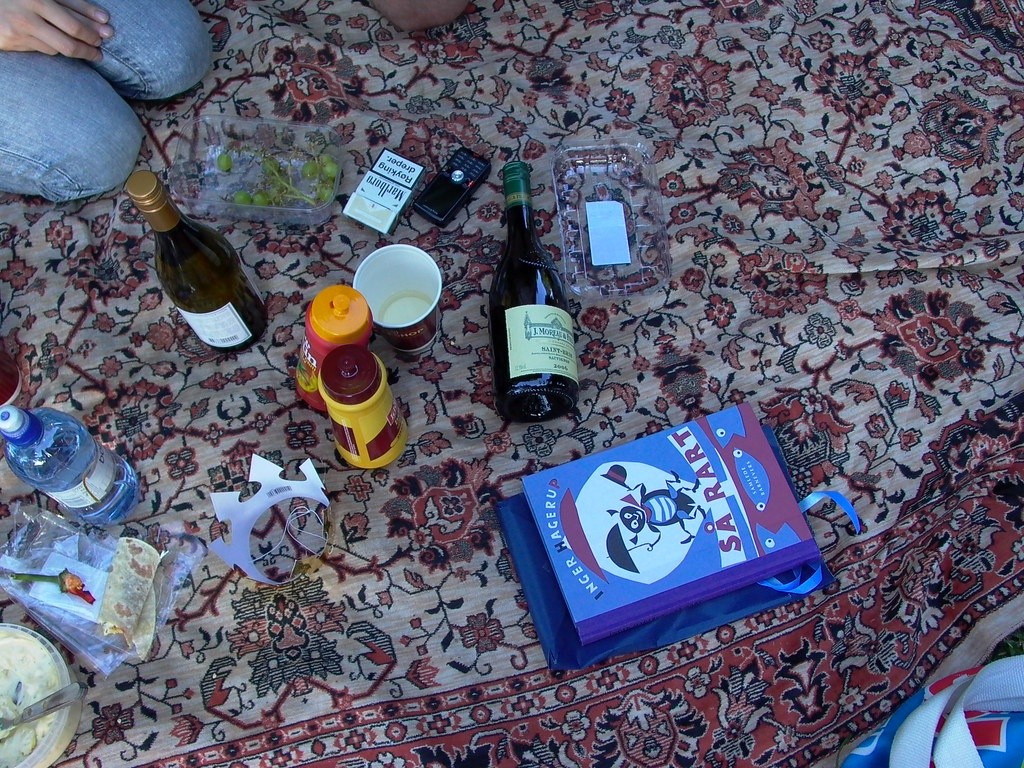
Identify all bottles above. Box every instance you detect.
[0,405,140,528]
[487,160,580,424]
[126,170,269,351]
[294,285,408,469]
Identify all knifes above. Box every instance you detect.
[0,682,88,730]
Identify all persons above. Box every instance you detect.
[0,0,469,202]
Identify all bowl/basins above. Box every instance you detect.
[0,623,83,768]
[167,113,345,226]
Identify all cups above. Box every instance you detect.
[0,346,22,408]
[352,245,441,352]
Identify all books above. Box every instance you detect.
[520,401,822,646]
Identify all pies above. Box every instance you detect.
[98,536,161,661]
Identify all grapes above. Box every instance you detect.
[217,152,337,206]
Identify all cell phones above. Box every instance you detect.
[413,146,492,230]
